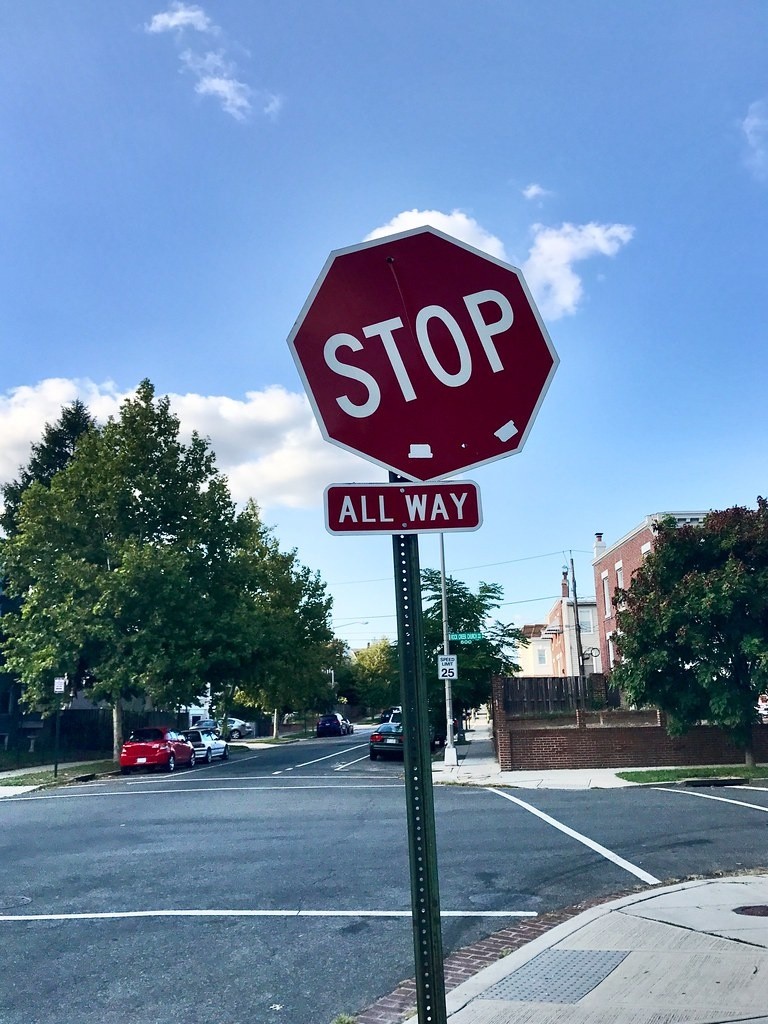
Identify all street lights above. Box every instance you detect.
[331,622,369,688]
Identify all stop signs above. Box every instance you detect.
[286,225,561,482]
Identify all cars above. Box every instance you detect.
[179,730,229,762]
[121,726,195,774]
[188,718,253,739]
[370,705,458,760]
[317,712,353,737]
